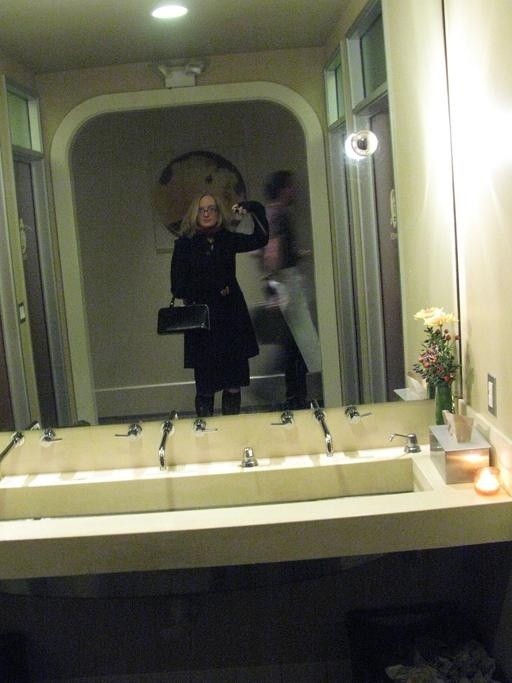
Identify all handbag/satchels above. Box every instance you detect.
[157,295,211,335]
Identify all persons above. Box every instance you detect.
[249,166,315,409]
[169,188,271,416]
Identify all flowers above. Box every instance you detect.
[418,311,462,385]
[411,305,445,382]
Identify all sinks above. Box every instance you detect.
[0,445,512,581]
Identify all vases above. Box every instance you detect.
[424,381,434,399]
[433,383,454,427]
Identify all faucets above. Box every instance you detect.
[168,410,179,420]
[313,409,334,457]
[0,432,25,464]
[157,421,176,472]
[388,432,421,452]
[310,399,320,409]
[24,420,41,431]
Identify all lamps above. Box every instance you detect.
[474,466,501,496]
[351,128,380,157]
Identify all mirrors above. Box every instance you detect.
[1,0,463,434]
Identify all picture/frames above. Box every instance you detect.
[148,140,259,254]
